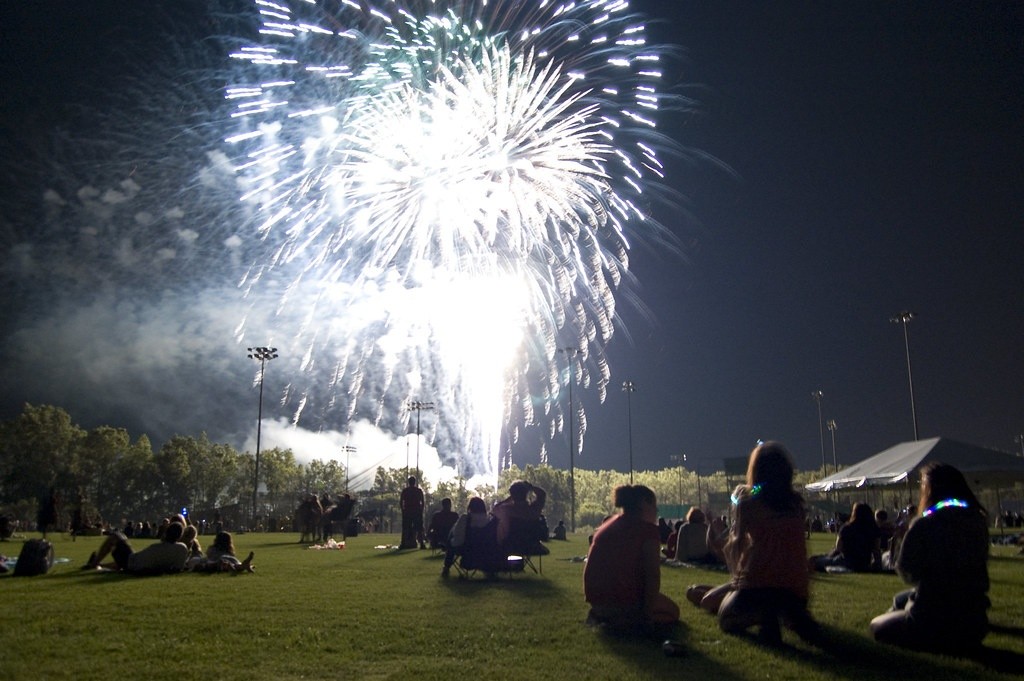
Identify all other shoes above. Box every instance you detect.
[420,542,427,549]
[441,567,450,575]
[87,551,98,569]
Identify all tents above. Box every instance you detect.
[805,437,1023,536]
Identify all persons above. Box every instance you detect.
[0,476,1024,615]
[718,440,825,647]
[870,463,992,655]
[586,485,680,639]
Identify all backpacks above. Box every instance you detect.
[13,538,55,576]
